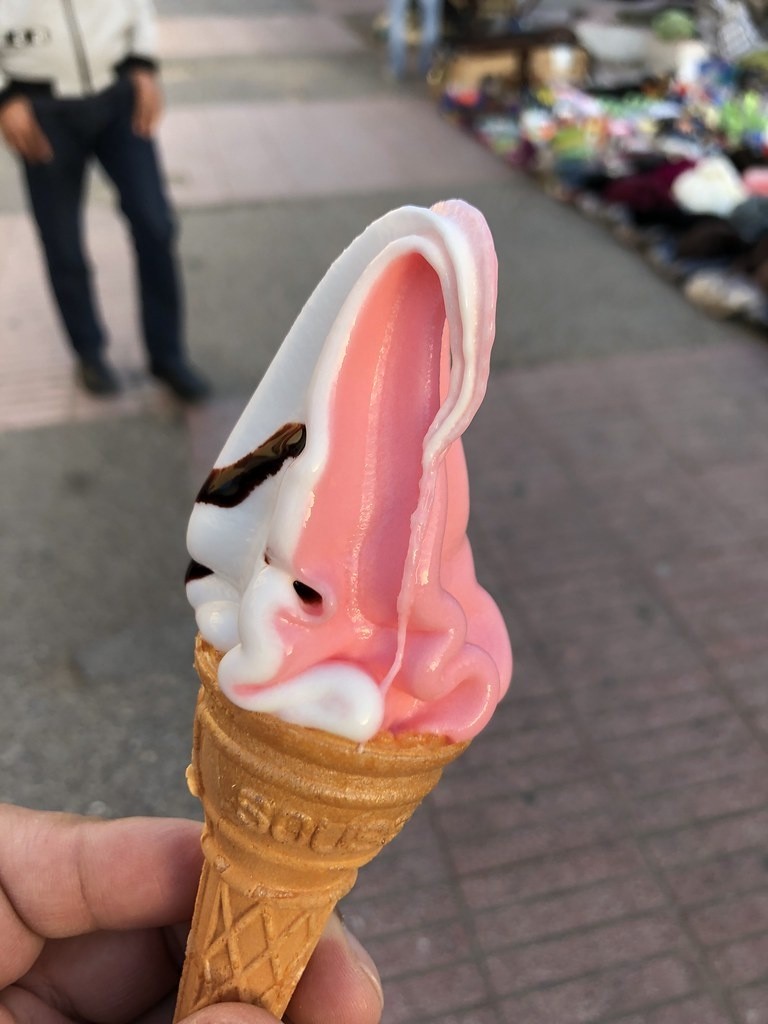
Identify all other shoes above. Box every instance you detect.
[150,357,208,403]
[81,355,117,396]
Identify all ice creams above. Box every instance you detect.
[170,197,514,1024]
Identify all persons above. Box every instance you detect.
[0,801,387,1024]
[0,0,214,406]
[382,0,441,87]
